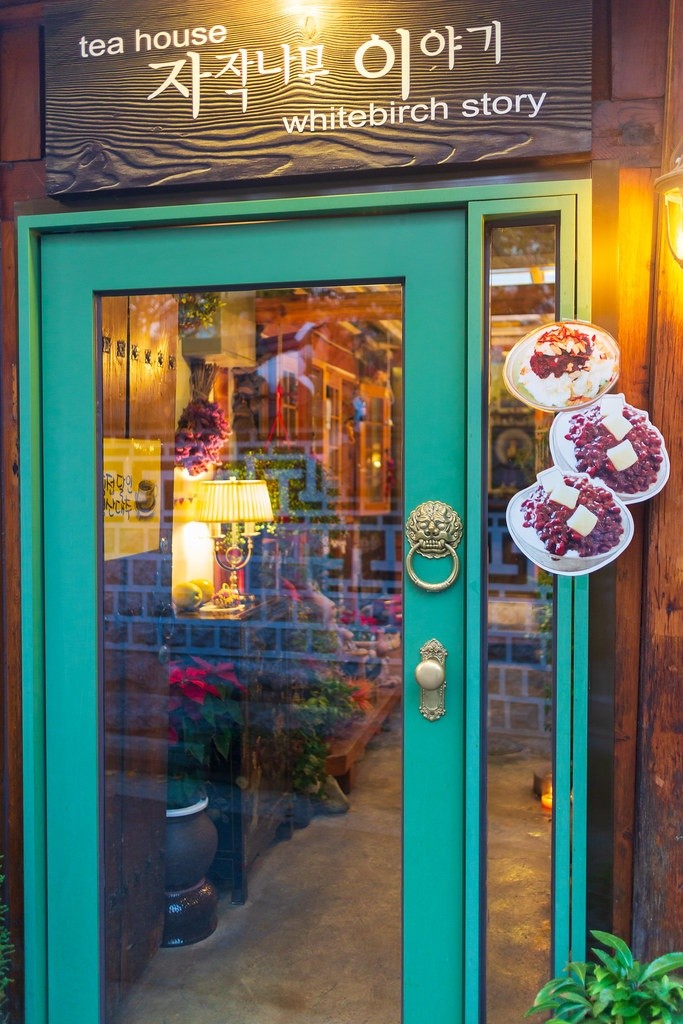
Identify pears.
[191,579,213,603]
[173,582,203,609]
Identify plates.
[506,480,635,577]
[503,321,620,411]
[549,398,670,506]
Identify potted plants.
[215,382,351,552]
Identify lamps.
[649,140,682,269]
[191,476,276,604]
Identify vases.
[177,317,200,335]
[164,791,219,892]
[354,641,377,672]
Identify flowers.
[176,292,227,329]
[166,655,251,810]
[174,360,234,476]
[340,610,376,640]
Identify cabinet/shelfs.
[268,350,396,533]
[170,596,298,907]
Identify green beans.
[522,479,623,555]
[565,406,662,493]
